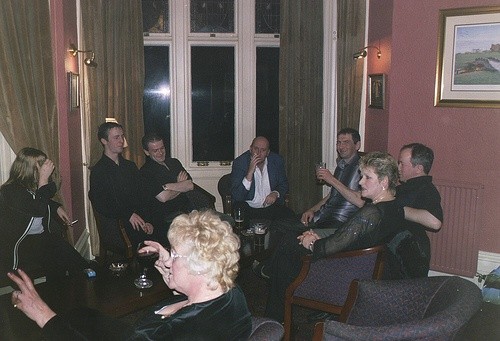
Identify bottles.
[317,162,326,184]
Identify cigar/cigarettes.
[254,153,257,156]
[302,222,308,223]
[69,220,78,226]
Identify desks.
[233,229,282,318]
[11,254,183,329]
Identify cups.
[139,273,152,288]
[254,223,267,234]
[235,208,246,222]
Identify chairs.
[311,275,484,341]
[0,209,70,292]
[282,242,393,341]
[217,172,290,231]
[244,315,285,341]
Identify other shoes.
[255,264,273,278]
[309,312,332,321]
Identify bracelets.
[164,184,168,190]
[309,240,315,252]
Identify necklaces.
[372,195,393,203]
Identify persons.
[0,122,443,324]
[7,210,252,341]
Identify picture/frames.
[432,5,500,109]
[67,72,80,114]
[367,72,387,110]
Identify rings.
[14,303,17,308]
[17,295,19,300]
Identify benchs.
[88,164,215,260]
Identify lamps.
[353,46,382,61]
[68,44,98,69]
